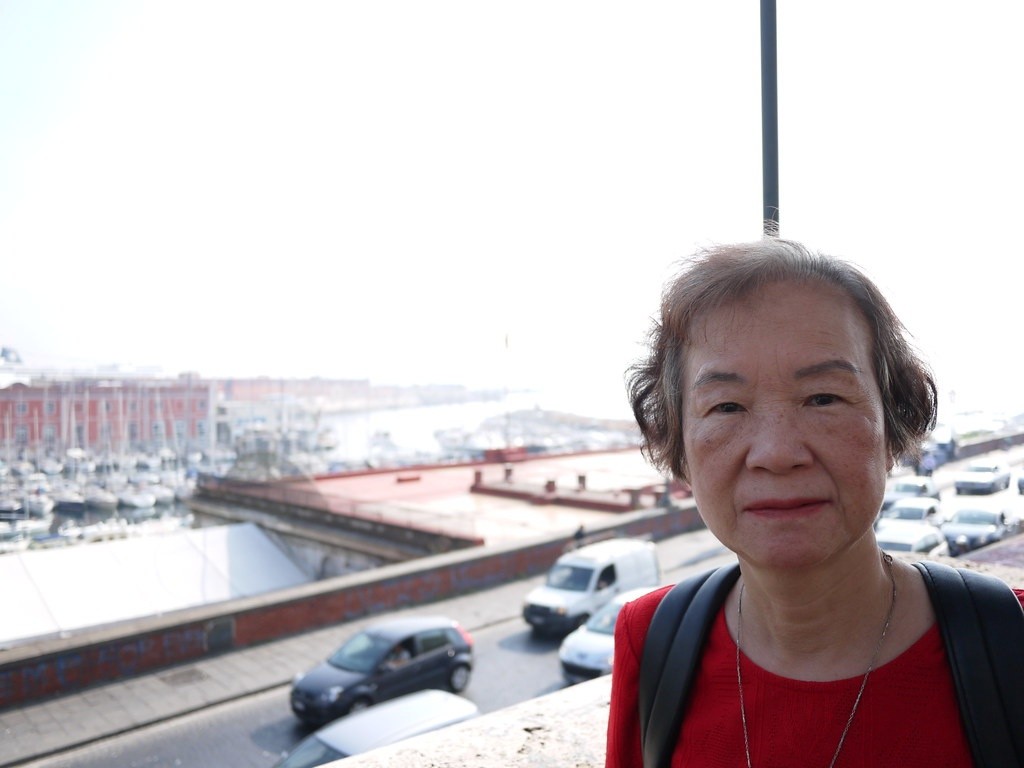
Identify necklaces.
[736,546,899,766]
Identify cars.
[875,451,1024,562]
[557,586,657,682]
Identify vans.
[523,540,661,635]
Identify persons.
[388,644,411,666]
[605,236,1024,768]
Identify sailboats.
[0,402,559,528]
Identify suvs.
[276,688,481,768]
[291,616,477,730]
